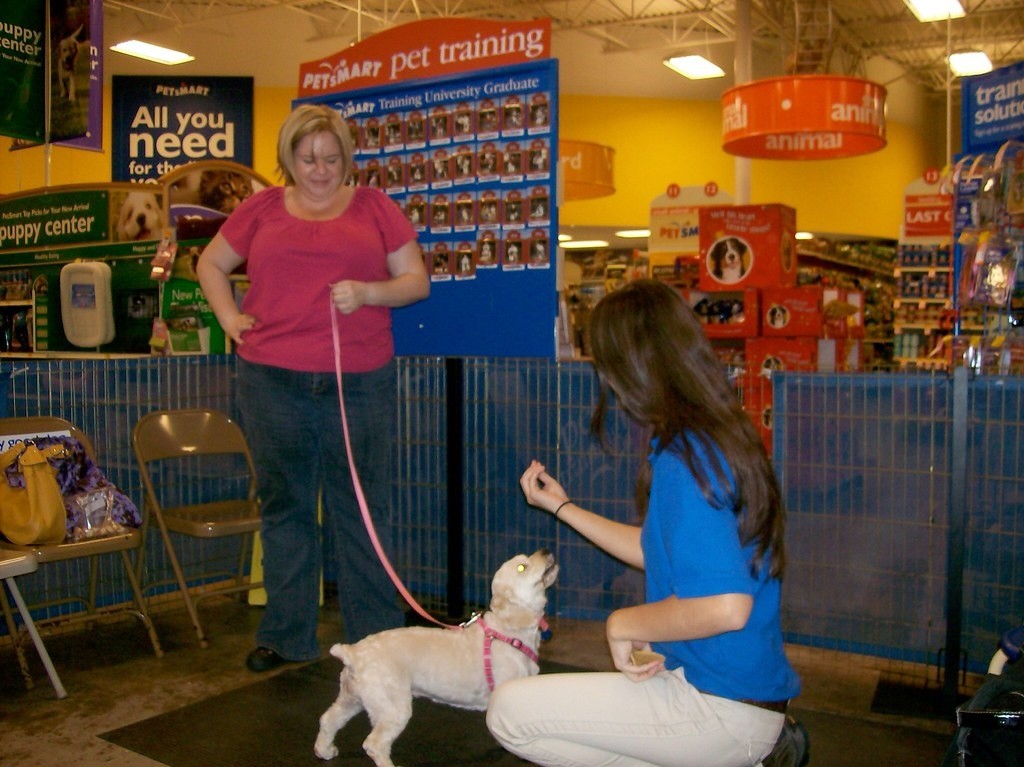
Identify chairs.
[0,549,70,697]
[133,409,261,650]
[0,414,162,692]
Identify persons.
[485,280,810,767]
[196,104,430,674]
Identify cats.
[169,169,254,216]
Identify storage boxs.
[653,200,865,467]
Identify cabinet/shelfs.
[888,242,1019,376]
[795,243,897,370]
[564,242,646,361]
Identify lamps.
[663,47,728,80]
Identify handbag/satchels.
[2,437,143,545]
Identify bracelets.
[554,500,573,516]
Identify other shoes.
[245,647,287,673]
[764,715,811,767]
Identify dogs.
[769,307,786,326]
[116,191,163,242]
[762,407,774,426]
[711,237,747,283]
[313,548,561,767]
[765,357,780,375]
